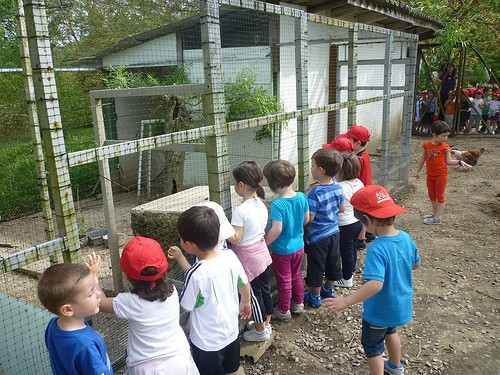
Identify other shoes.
[365,234,376,240]
[421,214,442,224]
[354,240,366,250]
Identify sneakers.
[383,358,404,375]
[320,283,337,298]
[290,301,304,314]
[334,274,353,287]
[272,305,291,322]
[243,326,270,341]
[246,320,273,334]
[303,292,322,308]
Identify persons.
[217,160,274,343]
[84,235,201,375]
[170,207,252,375]
[337,124,378,248]
[322,137,353,156]
[164,201,234,271]
[262,159,310,321]
[322,186,421,375]
[415,121,475,224]
[303,149,346,306]
[415,83,500,137]
[333,156,366,289]
[37,261,116,375]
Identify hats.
[193,201,236,240]
[120,236,169,281]
[466,88,473,96]
[322,137,353,153]
[474,88,484,95]
[350,185,408,219]
[338,125,371,142]
[463,90,469,95]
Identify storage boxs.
[87,229,107,246]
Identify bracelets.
[458,160,462,166]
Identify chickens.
[451,147,485,166]
